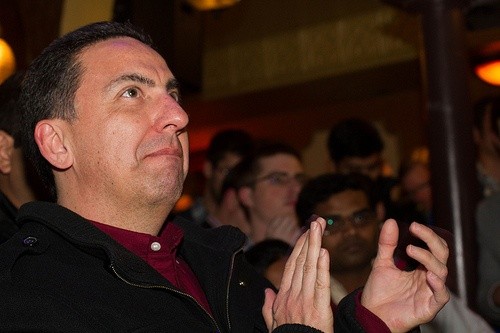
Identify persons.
[198,91,500,333]
[0,61,58,249]
[0,19,451,333]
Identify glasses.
[250,170,306,189]
[325,210,375,234]
